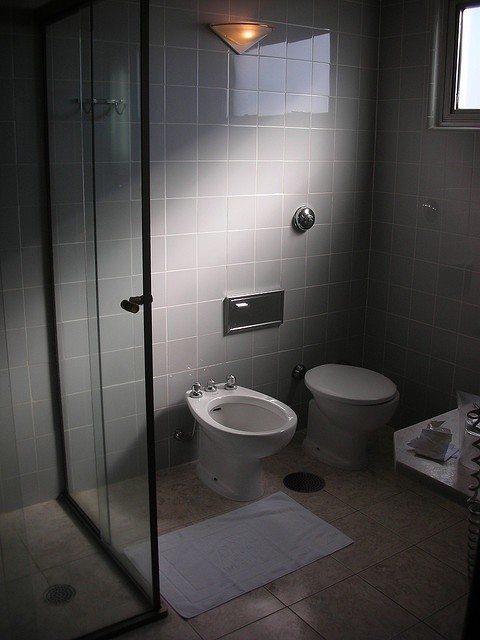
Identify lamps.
[210,19,273,55]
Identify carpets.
[123,489,355,619]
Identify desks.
[393,400,477,514]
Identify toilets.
[175,374,298,501]
[299,363,401,471]
